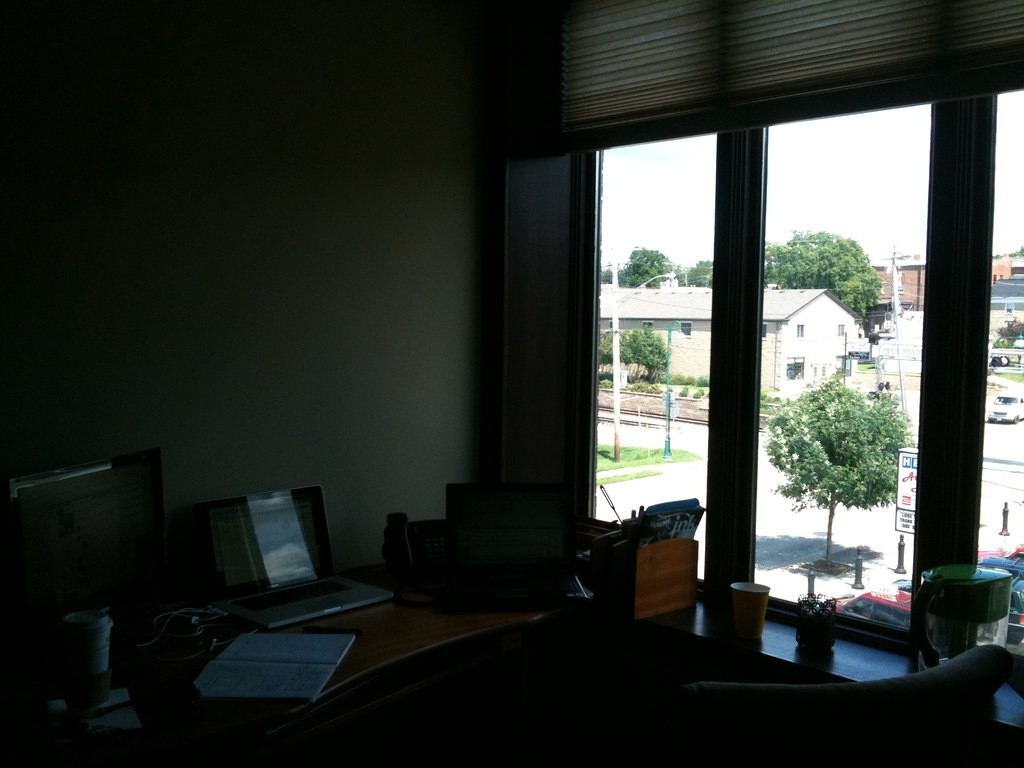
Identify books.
[194,633,355,701]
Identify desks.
[0,567,596,768]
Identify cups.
[729,582,770,639]
[59,610,114,674]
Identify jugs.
[910,564,1013,672]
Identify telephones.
[378,512,454,585]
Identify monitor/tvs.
[0,447,171,636]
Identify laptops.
[441,481,578,610]
[194,485,403,629]
